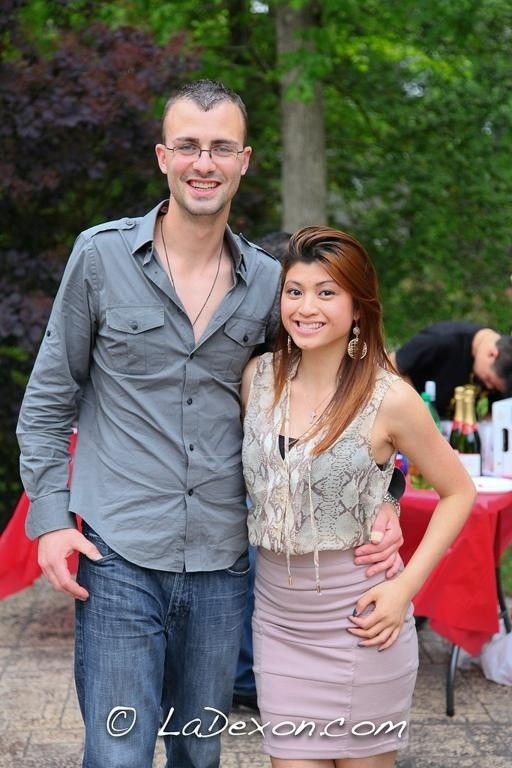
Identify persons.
[229,221,482,768]
[377,318,511,427]
[11,73,406,768]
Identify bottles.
[395,380,482,489]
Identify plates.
[469,476,512,494]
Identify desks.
[389,462,512,718]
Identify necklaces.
[156,214,227,330]
[298,372,338,424]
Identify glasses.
[164,142,244,162]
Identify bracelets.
[383,490,401,518]
